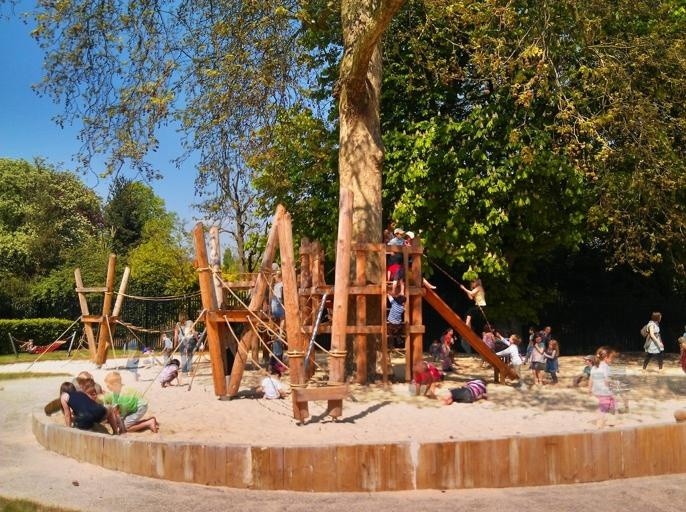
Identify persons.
[569,355,594,386]
[413,280,559,404]
[60,367,159,433]
[384,224,438,353]
[268,264,290,375]
[123,311,203,388]
[678,337,685,372]
[587,346,618,414]
[17,339,67,354]
[641,311,667,369]
